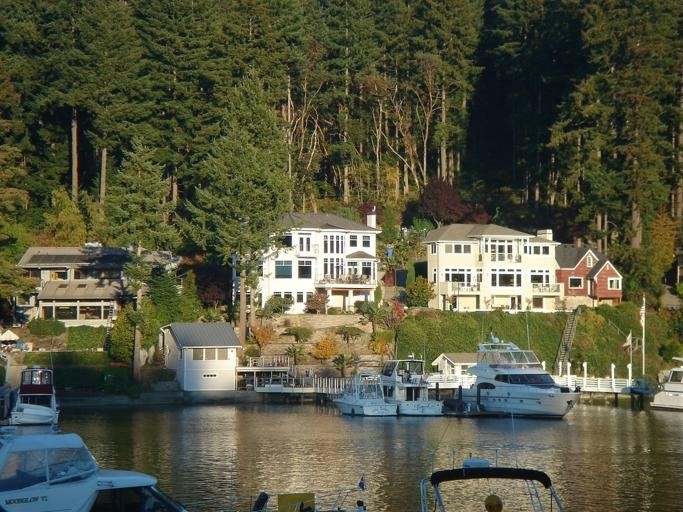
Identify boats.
[9,367,61,426]
[330,324,583,421]
[0,432,188,512]
[651,364,682,410]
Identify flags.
[622,333,632,346]
[639,299,645,327]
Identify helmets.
[485,495,502,512]
